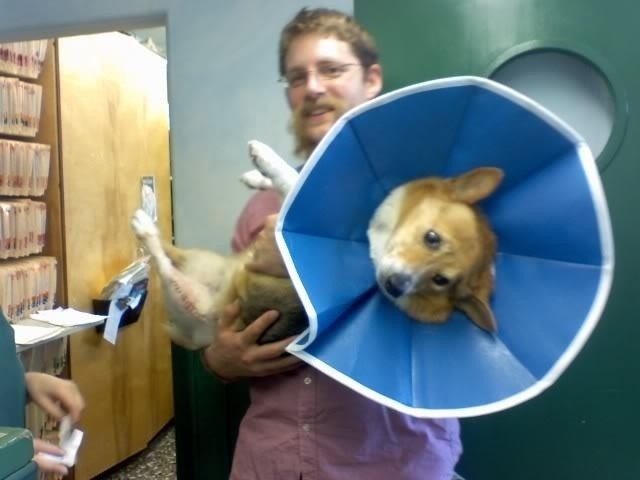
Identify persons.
[1,310,84,476]
[203,7,467,479]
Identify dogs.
[129,138,504,347]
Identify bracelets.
[204,351,233,382]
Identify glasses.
[277,60,365,89]
